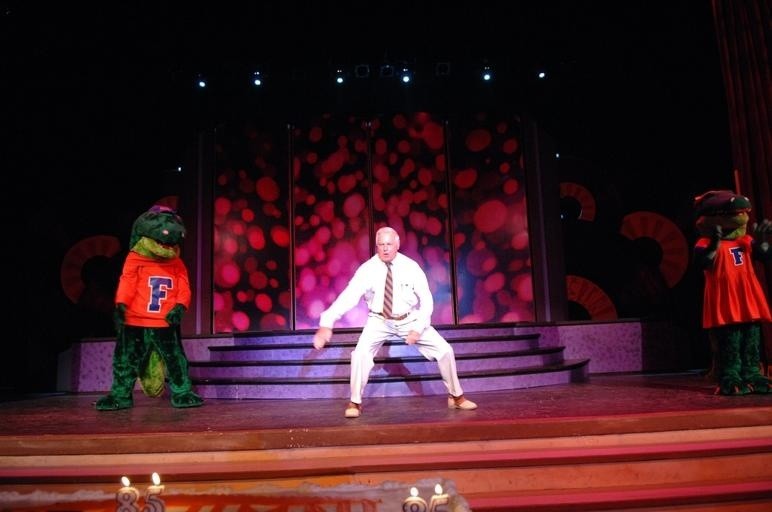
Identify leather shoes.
[447,393,478,410]
[344,402,362,418]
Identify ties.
[382,262,393,319]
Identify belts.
[373,312,409,320]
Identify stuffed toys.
[692,190,771,397]
[93,207,205,412]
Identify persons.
[313,226,478,419]
